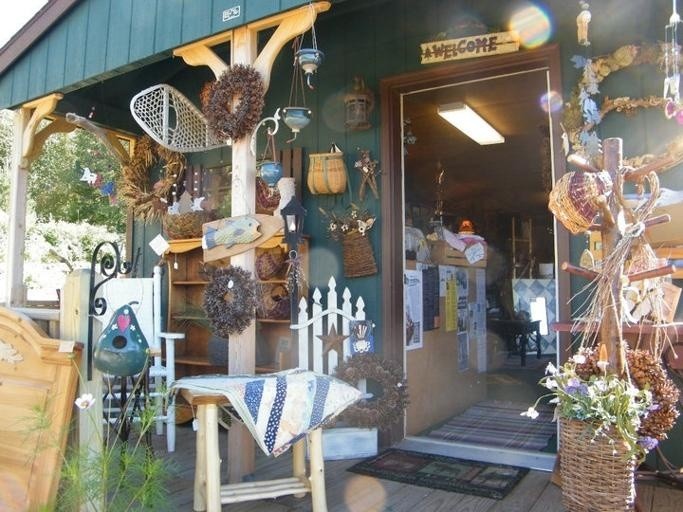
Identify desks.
[489,320,541,367]
[553,322,683,368]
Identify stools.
[180,388,328,512]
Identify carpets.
[427,400,556,452]
[345,448,530,501]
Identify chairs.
[102,265,185,452]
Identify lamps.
[437,102,505,146]
[344,75,375,131]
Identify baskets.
[307,153,347,195]
[558,415,636,512]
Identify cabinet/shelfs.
[162,234,310,424]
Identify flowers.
[520,355,659,464]
[319,202,376,242]
